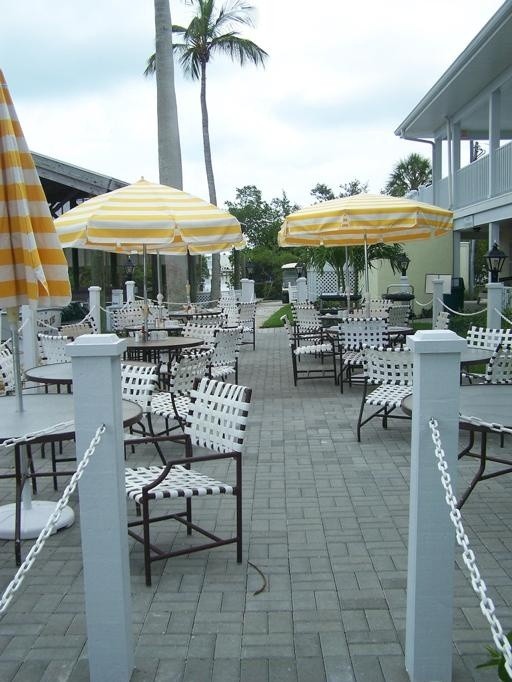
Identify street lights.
[294,256,314,297]
[391,251,413,302]
[474,239,511,331]
[242,258,258,298]
[119,255,138,302]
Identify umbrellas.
[1,66,74,414]
[272,186,456,316]
[51,174,248,334]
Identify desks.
[2,394,143,541]
[23,358,158,458]
[398,383,511,516]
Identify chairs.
[279,298,454,394]
[126,375,254,587]
[32,294,259,393]
[355,324,512,442]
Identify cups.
[149,331,168,340]
[338,310,349,318]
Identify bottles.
[154,317,159,328]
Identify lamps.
[123,256,136,280]
[483,241,508,282]
[395,254,410,276]
